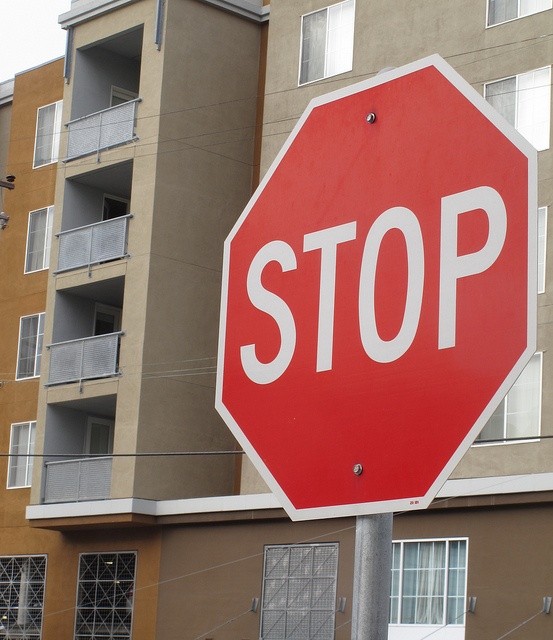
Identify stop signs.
[212,53,538,524]
